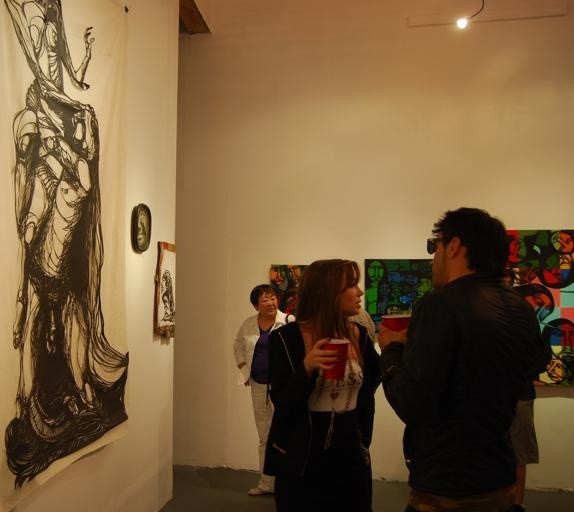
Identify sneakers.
[248,488,266,495]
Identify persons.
[233,259,377,511]
[136,205,149,253]
[10,21,104,425]
[378,208,553,511]
[160,271,176,325]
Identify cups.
[382,315,411,335]
[322,339,349,383]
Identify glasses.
[427,238,450,255]
